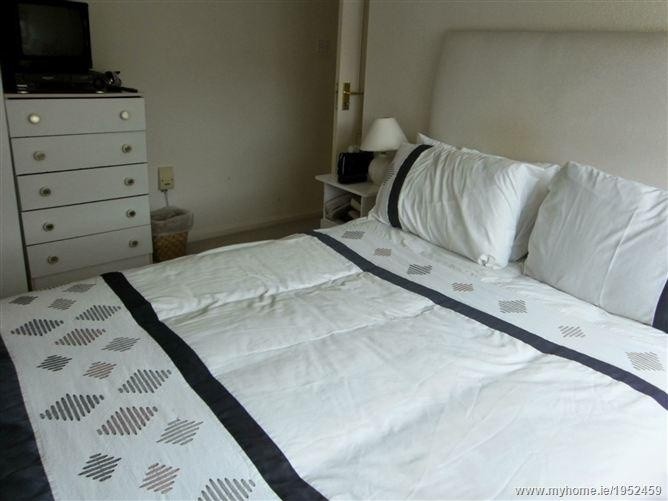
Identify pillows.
[367,130,559,272]
[521,162,668,335]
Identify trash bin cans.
[151,206,194,263]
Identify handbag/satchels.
[325,194,351,221]
[337,151,374,184]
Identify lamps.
[358,116,409,187]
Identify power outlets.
[158,166,174,194]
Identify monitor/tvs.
[0,0,93,93]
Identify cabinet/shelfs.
[1,92,154,301]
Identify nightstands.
[315,171,381,229]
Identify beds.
[0,212,668,500]
